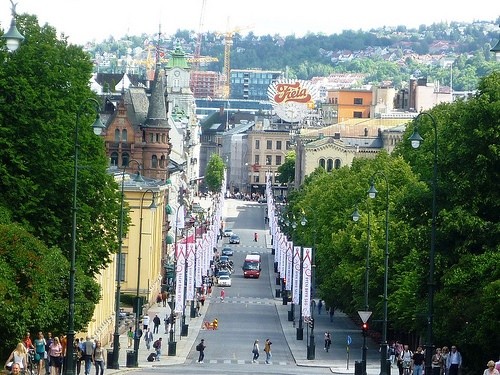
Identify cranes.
[131,43,220,81]
[211,22,258,101]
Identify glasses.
[490,366,494,367]
[451,348,455,349]
[54,339,57,341]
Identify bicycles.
[27,348,40,375]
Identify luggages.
[147,352,157,362]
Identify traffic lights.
[309,318,314,329]
[362,323,367,337]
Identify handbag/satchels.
[264,349,265,351]
[253,343,255,353]
[90,349,96,362]
[44,351,48,359]
[35,354,40,361]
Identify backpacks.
[153,340,159,348]
[196,343,201,351]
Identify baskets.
[403,361,410,369]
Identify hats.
[256,339,259,341]
[201,339,205,341]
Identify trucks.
[242,254,262,279]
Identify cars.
[217,275,232,287]
[229,235,240,245]
[221,247,235,256]
[251,251,261,262]
[217,256,231,278]
[223,230,234,237]
[258,197,267,203]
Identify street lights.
[167,202,194,356]
[291,211,304,340]
[108,159,146,370]
[284,212,295,321]
[181,190,220,337]
[130,189,158,368]
[406,113,439,375]
[351,198,369,375]
[265,177,288,306]
[300,206,315,360]
[63,98,107,375]
[366,172,392,375]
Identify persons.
[221,288,225,303]
[265,339,272,364]
[154,338,162,361]
[198,339,206,364]
[138,326,142,349]
[318,300,322,314]
[153,315,160,334]
[7,363,25,375]
[92,341,105,375]
[143,313,150,330]
[157,291,167,307]
[253,339,259,364]
[379,340,426,375]
[431,346,462,375]
[208,286,212,297]
[143,328,153,349]
[324,332,331,351]
[127,326,134,348]
[47,336,62,375]
[4,330,99,375]
[197,284,206,317]
[483,359,500,375]
[164,314,169,334]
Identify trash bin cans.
[125,349,136,367]
[276,277,279,285]
[106,349,113,368]
[283,297,287,305]
[296,328,304,339]
[272,249,274,255]
[73,352,77,375]
[274,263,276,268]
[275,289,280,297]
[168,342,177,356]
[355,361,363,375]
[307,345,315,358]
[380,359,392,375]
[265,219,268,224]
[194,308,196,316]
[185,325,188,336]
[288,311,292,320]
[210,260,213,267]
[196,287,200,292]
[207,270,210,276]
